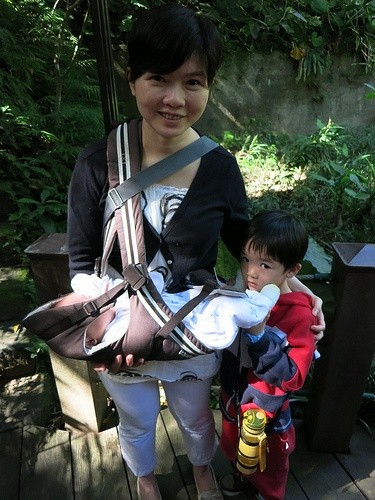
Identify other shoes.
[218,469,266,500]
[192,463,224,500]
[136,476,162,500]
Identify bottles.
[236,408,269,475]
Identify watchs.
[244,329,265,345]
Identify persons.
[45,273,281,359]
[60,3,325,500]
[219,206,320,500]
